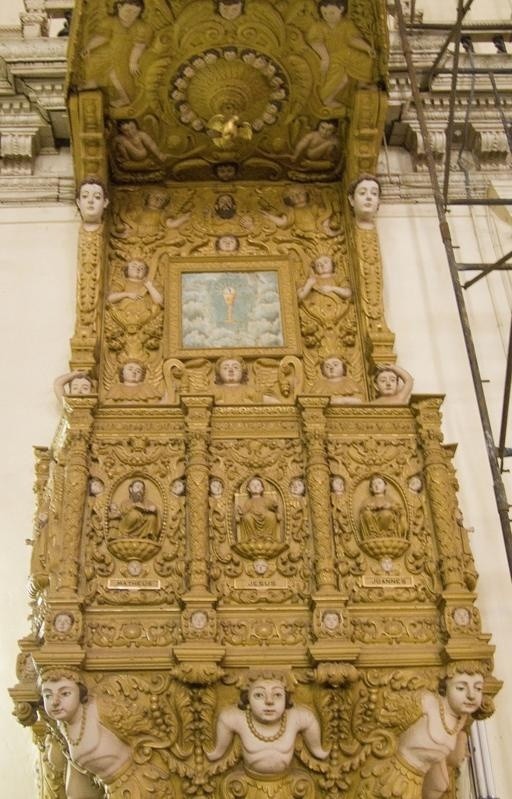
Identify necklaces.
[435,693,461,736]
[244,705,288,743]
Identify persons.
[21,666,158,797]
[59,706,91,745]
[358,657,485,799]
[193,668,330,798]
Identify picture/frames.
[159,250,301,364]
